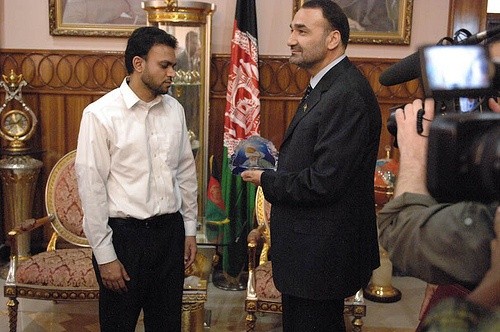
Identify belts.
[108,211,179,224]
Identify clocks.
[0,69,43,267]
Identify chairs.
[6,149,208,332]
[245,185,367,332]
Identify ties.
[298,82,313,107]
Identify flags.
[223,0,261,142]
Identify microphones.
[381,26,499,85]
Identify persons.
[74,27,198,332]
[378,97,500,332]
[171,31,199,129]
[241,0,383,332]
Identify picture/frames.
[293,0,414,46]
[48,0,159,37]
